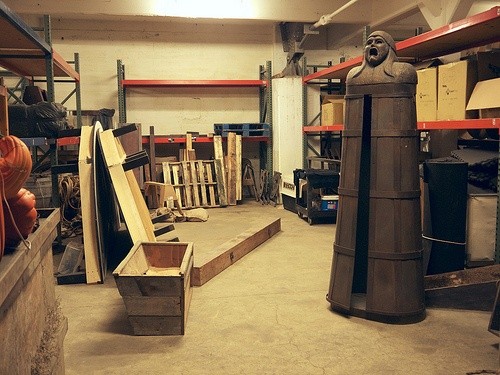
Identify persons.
[347,31,418,84]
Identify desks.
[0,207,68,375]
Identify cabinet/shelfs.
[301,6,500,270]
[116,59,273,195]
[0,0,81,246]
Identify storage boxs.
[321,194,340,210]
[321,94,345,126]
[416,47,500,121]
[281,177,307,213]
[112,242,194,336]
